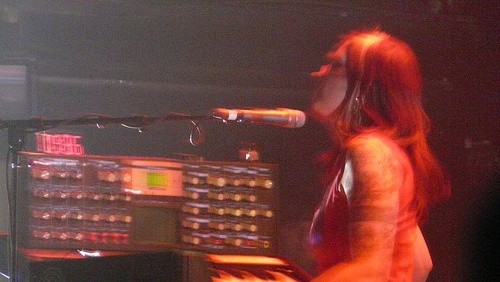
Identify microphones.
[212,108,306,129]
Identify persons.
[308,30,450,282]
